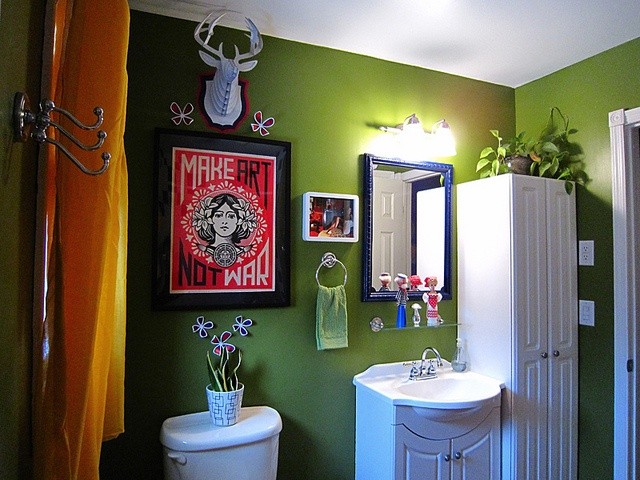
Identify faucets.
[418,345,443,377]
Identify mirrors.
[361,152,453,302]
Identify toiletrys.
[450,335,469,372]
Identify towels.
[315,284,348,350]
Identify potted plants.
[206,344,245,427]
[475,107,577,196]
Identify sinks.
[353,358,506,422]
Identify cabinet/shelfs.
[457,175,578,480]
[355,393,503,480]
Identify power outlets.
[579,240,594,266]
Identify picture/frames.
[302,192,359,243]
[149,128,292,310]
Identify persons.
[395,273,410,329]
[422,276,443,322]
[192,193,257,256]
[324,204,334,228]
